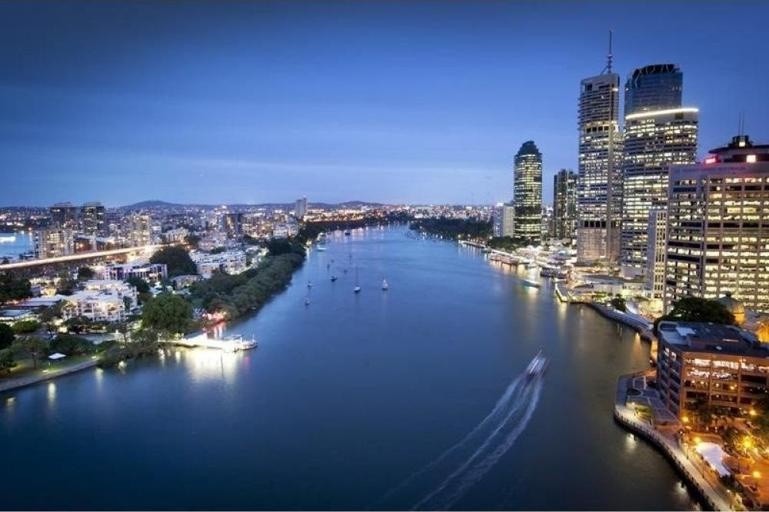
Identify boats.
[303,245,389,308]
[236,337,258,351]
[344,230,350,236]
[490,253,516,264]
[522,277,541,287]
[526,349,545,376]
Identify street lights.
[680,399,762,508]
